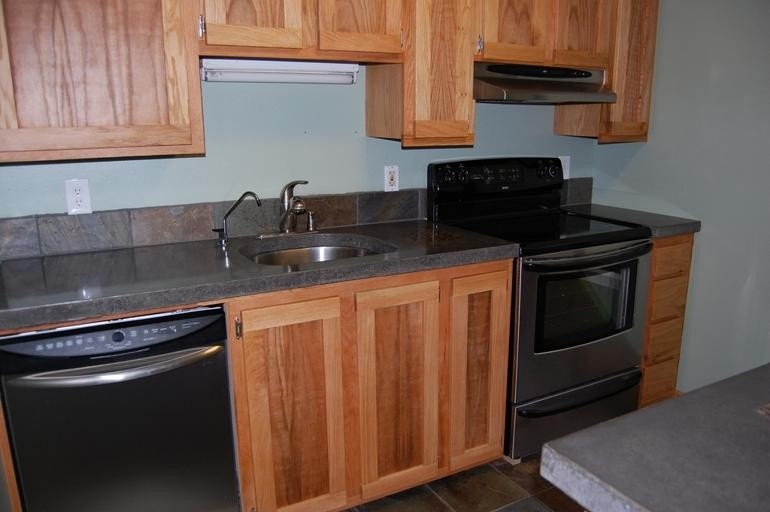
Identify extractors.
[473,60,619,104]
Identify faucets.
[278,180,309,235]
[222,191,262,245]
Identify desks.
[538,360,770,510]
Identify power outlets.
[382,164,403,193]
[65,177,93,216]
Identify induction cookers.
[444,210,650,258]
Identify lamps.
[200,56,359,87]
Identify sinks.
[237,233,399,266]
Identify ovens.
[503,240,652,464]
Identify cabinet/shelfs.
[639,232,694,408]
[366,1,475,149]
[230,259,514,512]
[555,1,661,145]
[1,1,206,176]
[201,1,405,68]
[476,1,614,71]
